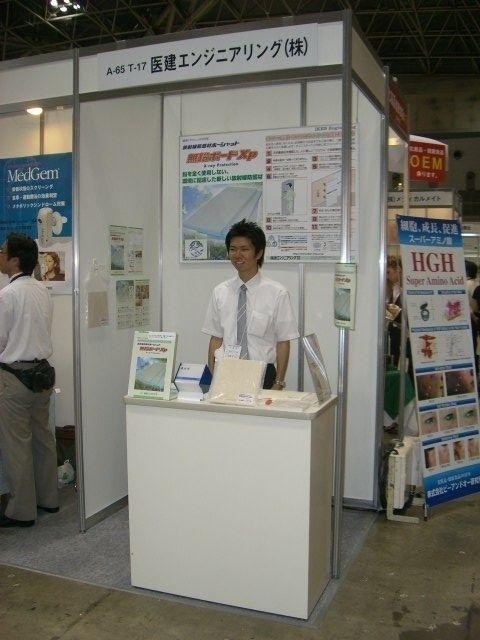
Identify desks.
[123,388,338,621]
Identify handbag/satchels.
[15,360,55,393]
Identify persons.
[384,253,416,383]
[416,367,480,468]
[200,217,301,394]
[470,284,479,337]
[41,252,65,281]
[0,230,62,528]
[464,259,479,354]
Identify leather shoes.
[37,505,59,513]
[0,514,34,527]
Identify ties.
[236,285,248,359]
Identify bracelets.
[273,379,287,388]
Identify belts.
[14,360,43,364]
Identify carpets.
[1,484,381,630]
